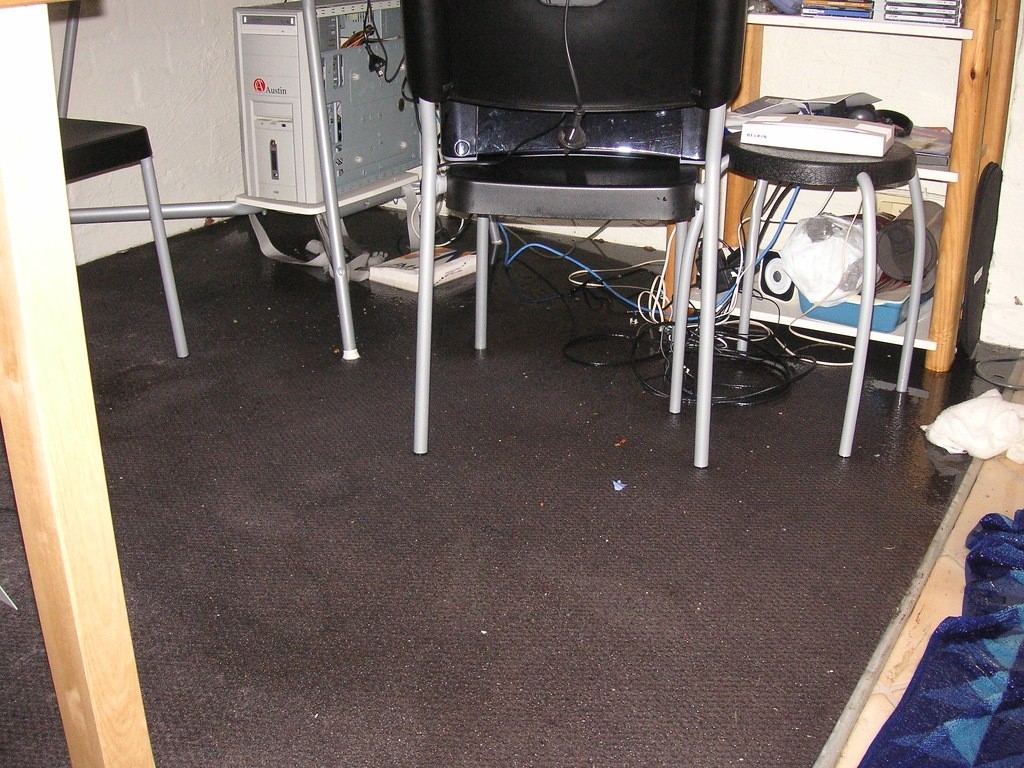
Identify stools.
[665,130,926,473]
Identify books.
[801,0,874,19]
[369,246,476,293]
[883,0,959,26]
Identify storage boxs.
[798,284,935,333]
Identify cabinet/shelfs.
[663,0,1024,372]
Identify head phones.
[848,104,914,138]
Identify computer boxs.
[232,0,421,206]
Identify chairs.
[401,0,746,457]
[57,0,191,358]
